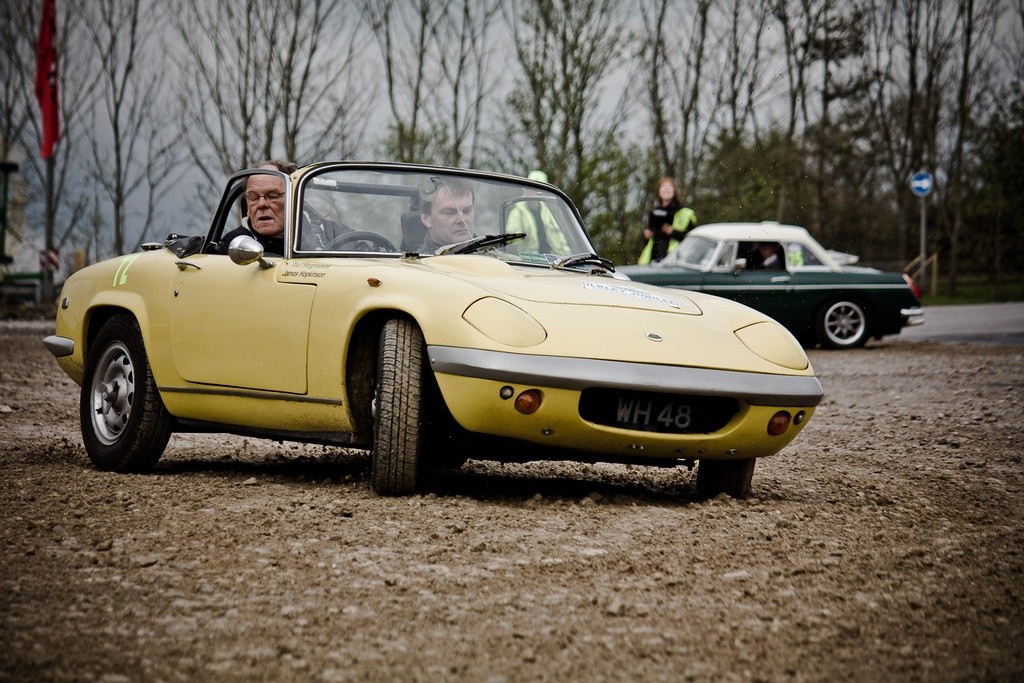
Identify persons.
[219,162,361,257]
[643,177,695,266]
[756,243,783,269]
[413,178,478,254]
[506,170,572,255]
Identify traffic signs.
[911,172,931,198]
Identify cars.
[614,222,923,347]
[42,162,826,498]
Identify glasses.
[245,190,285,201]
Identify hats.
[527,170,548,184]
